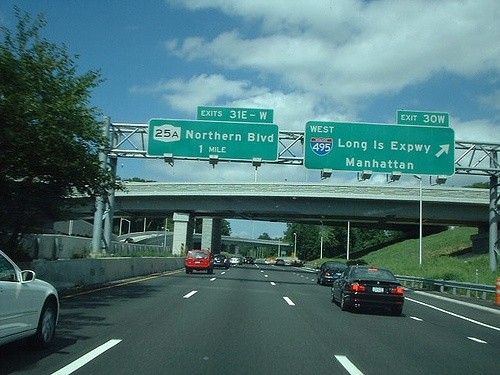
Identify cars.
[0,251,61,346]
[316,262,346,285]
[214,252,306,268]
[184,250,215,272]
[330,263,404,315]
